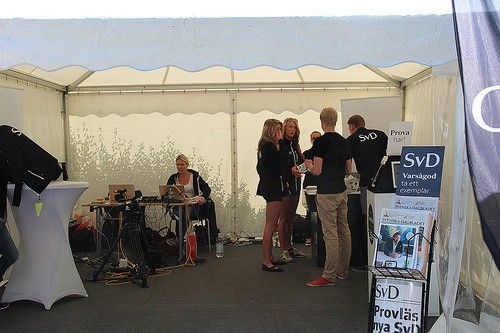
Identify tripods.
[93,205,158,288]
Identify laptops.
[109,183,140,201]
[159,184,194,201]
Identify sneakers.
[288,247,306,257]
[307,276,335,286]
[280,250,293,262]
[334,271,347,279]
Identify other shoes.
[271,259,287,265]
[304,238,311,246]
[0,302,9,310]
[262,264,284,272]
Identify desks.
[1,182,89,309]
[82,201,197,260]
[302,189,363,268]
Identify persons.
[304,106,352,287]
[278,118,306,262]
[0,214,19,309]
[256,119,304,272]
[346,114,388,273]
[166,155,219,256]
[303,131,322,177]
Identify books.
[396,145,445,197]
[387,121,412,156]
[371,195,439,279]
[372,278,423,333]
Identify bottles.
[216,233,224,258]
